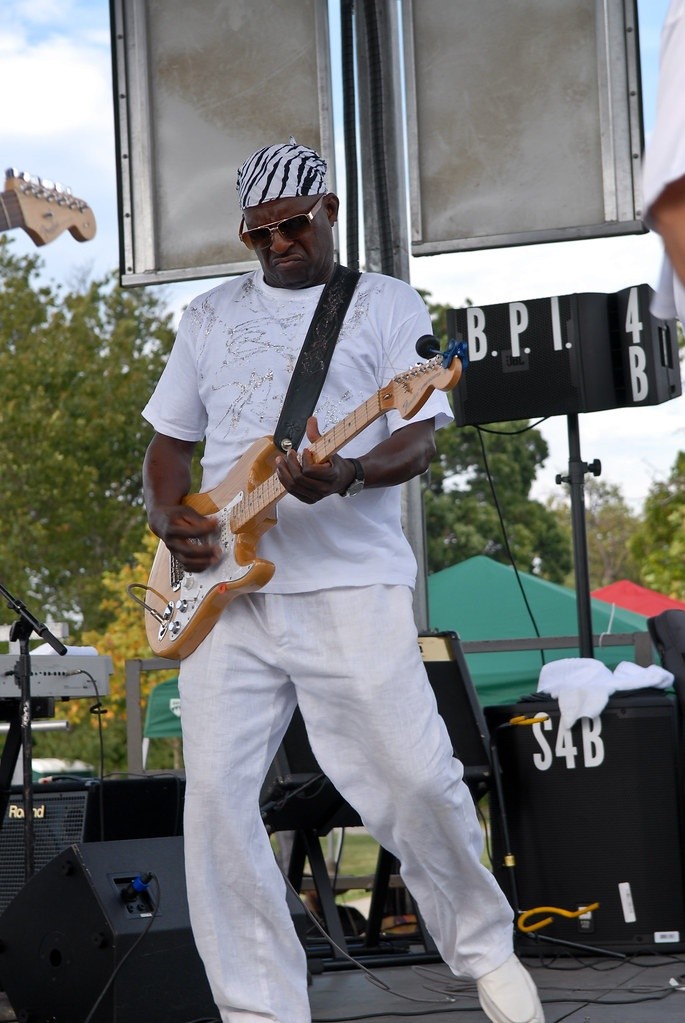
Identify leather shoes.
[476,954,545,1023]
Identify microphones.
[416,334,445,360]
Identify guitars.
[1,166,97,248]
[126,350,464,663]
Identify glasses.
[238,195,326,250]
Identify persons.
[139,137,547,1023]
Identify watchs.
[340,457,366,499]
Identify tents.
[143,556,675,821]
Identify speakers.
[446,282,680,427]
[0,772,223,1023]
[260,628,496,805]
[482,692,685,957]
